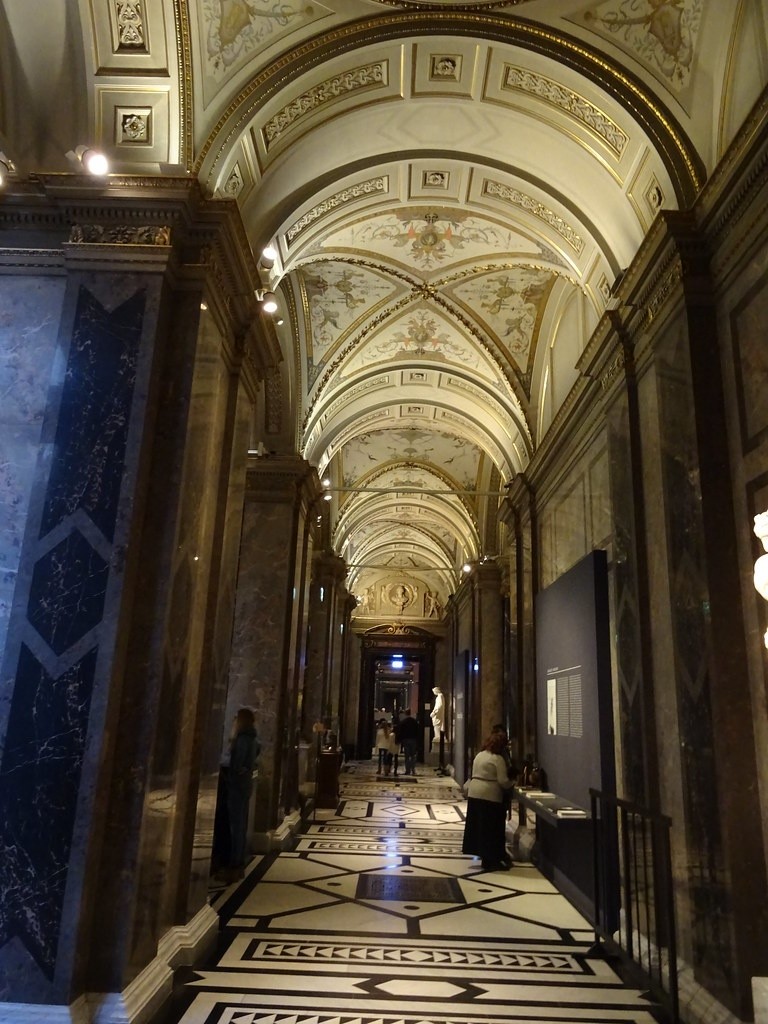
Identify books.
[517,785,587,819]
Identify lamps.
[463,555,489,571]
[259,246,281,316]
[66,143,110,180]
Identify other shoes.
[482,858,512,870]
[394,770,398,776]
[403,772,410,775]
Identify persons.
[462,725,518,871]
[430,687,443,743]
[214,709,260,882]
[374,706,421,777]
[314,723,335,748]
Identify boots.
[384,766,388,776]
[376,766,381,774]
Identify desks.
[315,751,344,808]
